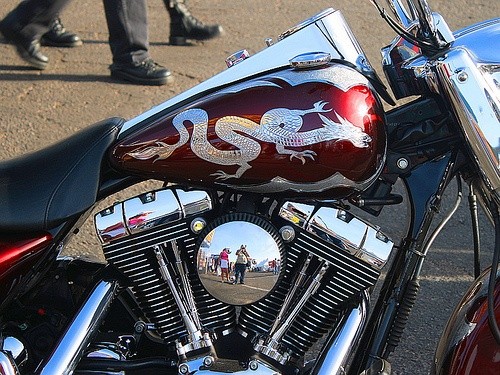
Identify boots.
[40,13,83,46]
[163,0,221,45]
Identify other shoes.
[227,280,231,282]
[222,281,225,282]
[233,282,236,284]
[240,282,244,284]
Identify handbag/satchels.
[216,265,222,276]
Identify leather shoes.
[0,21,49,69]
[112,58,173,85]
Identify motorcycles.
[0,0,500,375]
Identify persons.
[207,244,281,285]
[40,0,225,48]
[0,0,173,84]
